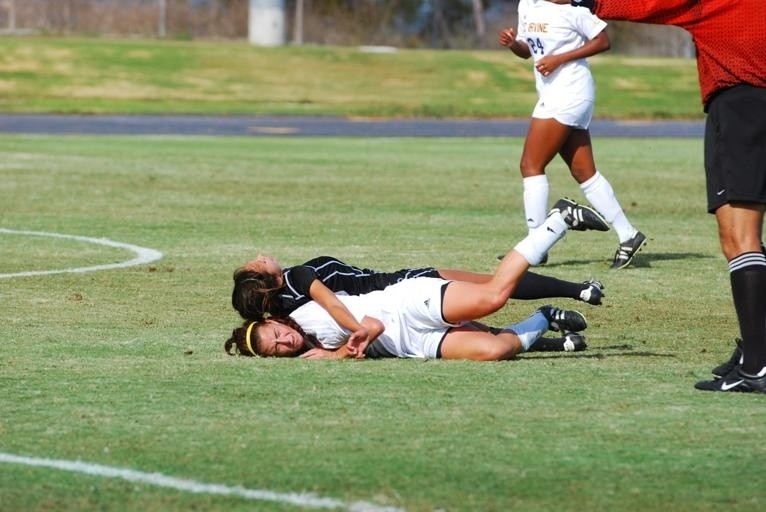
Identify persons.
[541,0,765,392]
[225,197,611,364]
[229,253,604,351]
[498,0,648,270]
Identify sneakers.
[695,336,766,391]
[579,276,604,303]
[532,305,588,352]
[610,231,647,270]
[547,195,609,232]
